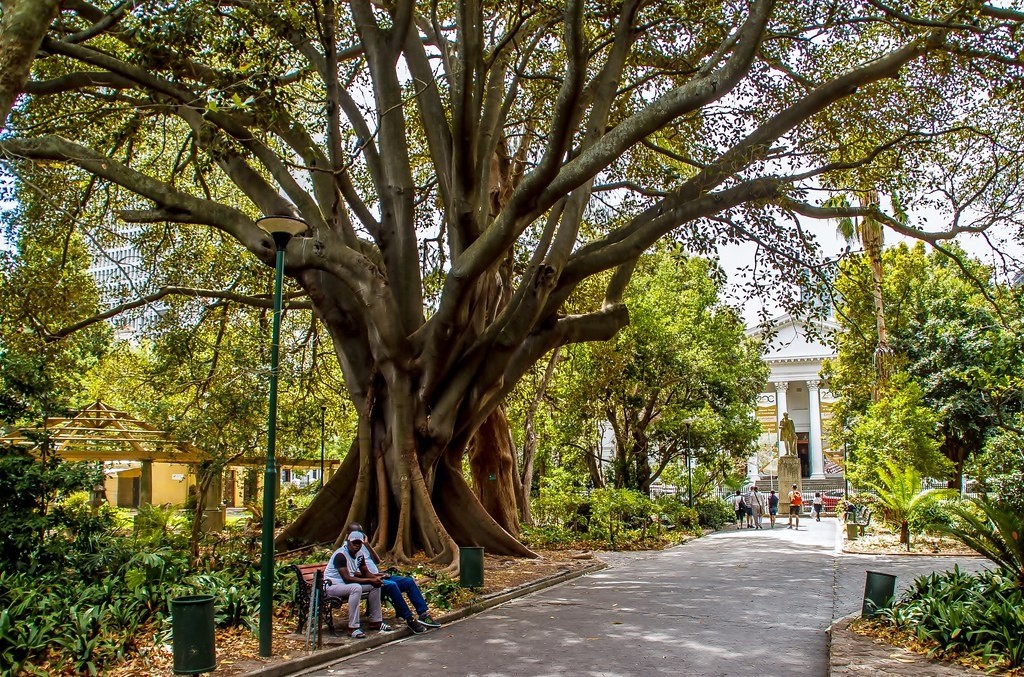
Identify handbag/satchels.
[760,506,765,515]
[769,507,778,513]
[811,508,817,516]
[739,497,747,513]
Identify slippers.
[369,623,392,630]
[348,629,365,638]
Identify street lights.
[320,406,327,487]
[254,215,311,659]
[682,419,695,508]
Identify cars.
[724,493,744,506]
[802,489,845,512]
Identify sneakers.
[417,613,442,627]
[407,618,427,634]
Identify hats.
[347,531,365,544]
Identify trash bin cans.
[862,570,896,619]
[458,546,485,588]
[847,524,857,540]
[171,595,216,675]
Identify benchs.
[287,563,401,634]
[845,505,874,536]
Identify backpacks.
[791,492,803,505]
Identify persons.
[768,489,778,528]
[750,486,765,529]
[322,531,391,638]
[344,522,441,634]
[744,486,755,528]
[813,492,822,521]
[733,490,746,529]
[288,499,297,509]
[779,412,795,456]
[788,484,802,530]
